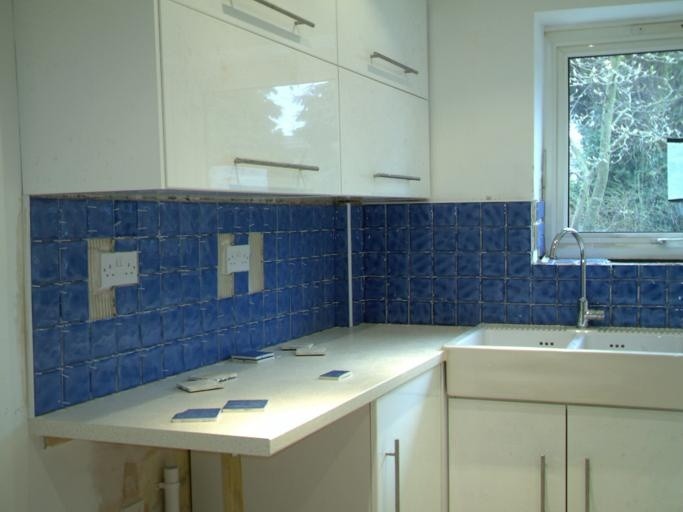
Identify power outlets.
[102,252,138,287]
[226,244,250,274]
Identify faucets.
[549,228,605,329]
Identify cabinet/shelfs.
[447,397,683,511]
[190,362,443,511]
[15,0,432,202]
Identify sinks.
[444,324,578,353]
[573,326,683,359]
